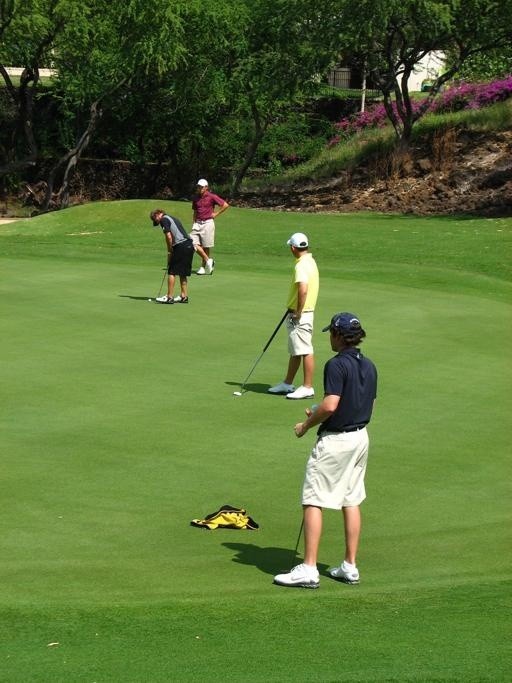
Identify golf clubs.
[278,519,304,574]
[233,310,289,395]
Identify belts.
[287,306,317,314]
[322,423,372,435]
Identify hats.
[151,208,164,226]
[321,312,362,333]
[286,231,309,249]
[196,179,209,187]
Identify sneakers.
[267,381,295,394]
[285,384,315,400]
[328,562,362,586]
[155,295,189,304]
[274,563,320,589]
[196,258,215,275]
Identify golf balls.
[311,405,319,412]
[148,299,152,302]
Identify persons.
[273,311,377,588]
[149,206,194,304]
[266,231,320,399]
[186,177,230,275]
